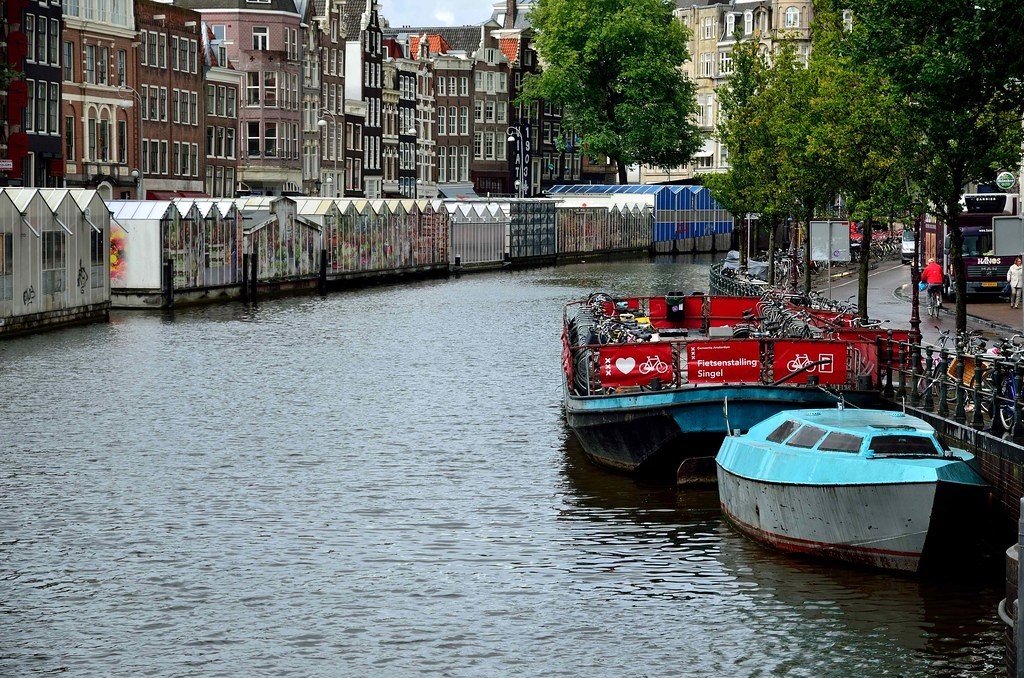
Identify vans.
[902,230,922,264]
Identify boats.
[713,403,992,579]
[561,291,886,487]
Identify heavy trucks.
[921,193,1024,302]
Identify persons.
[1006,257,1024,309]
[921,258,943,316]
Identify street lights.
[316,108,337,198]
[507,126,524,198]
[409,117,426,198]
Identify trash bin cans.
[665,291,686,323]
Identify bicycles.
[922,280,942,319]
[565,294,675,392]
[723,236,1024,433]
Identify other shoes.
[1015,307,1018,309]
[1011,302,1013,309]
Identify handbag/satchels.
[1002,282,1011,294]
[918,281,928,291]
